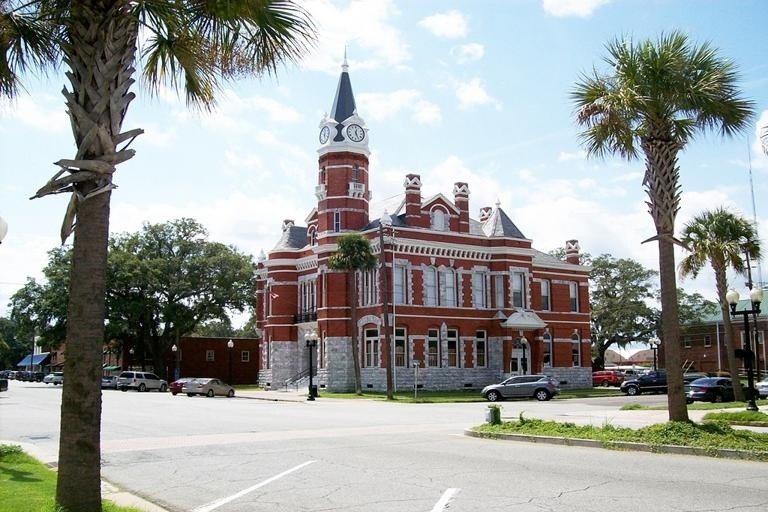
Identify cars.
[0,370,235,398]
[592,367,768,404]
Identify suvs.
[480,374,562,402]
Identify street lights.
[647,335,661,370]
[128,347,134,371]
[171,343,177,380]
[518,335,528,375]
[226,338,235,385]
[303,329,318,401]
[725,283,765,412]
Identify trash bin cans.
[0,379,8,391]
[307,385,317,400]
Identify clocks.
[319,126,330,144]
[346,123,365,142]
